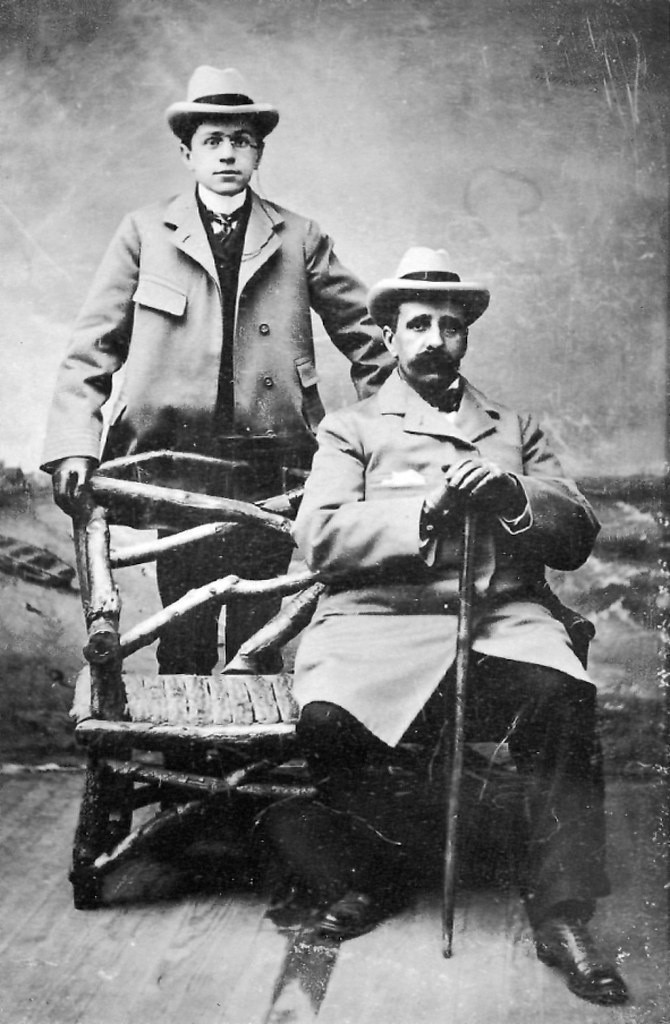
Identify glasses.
[192,137,259,151]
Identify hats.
[166,65,278,140]
[366,247,491,332]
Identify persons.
[41,64,394,674]
[297,244,626,1005]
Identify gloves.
[422,485,489,540]
[447,457,516,511]
[51,455,94,517]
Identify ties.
[211,212,234,245]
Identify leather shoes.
[319,881,416,935]
[537,916,629,1002]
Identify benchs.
[63,448,614,916]
